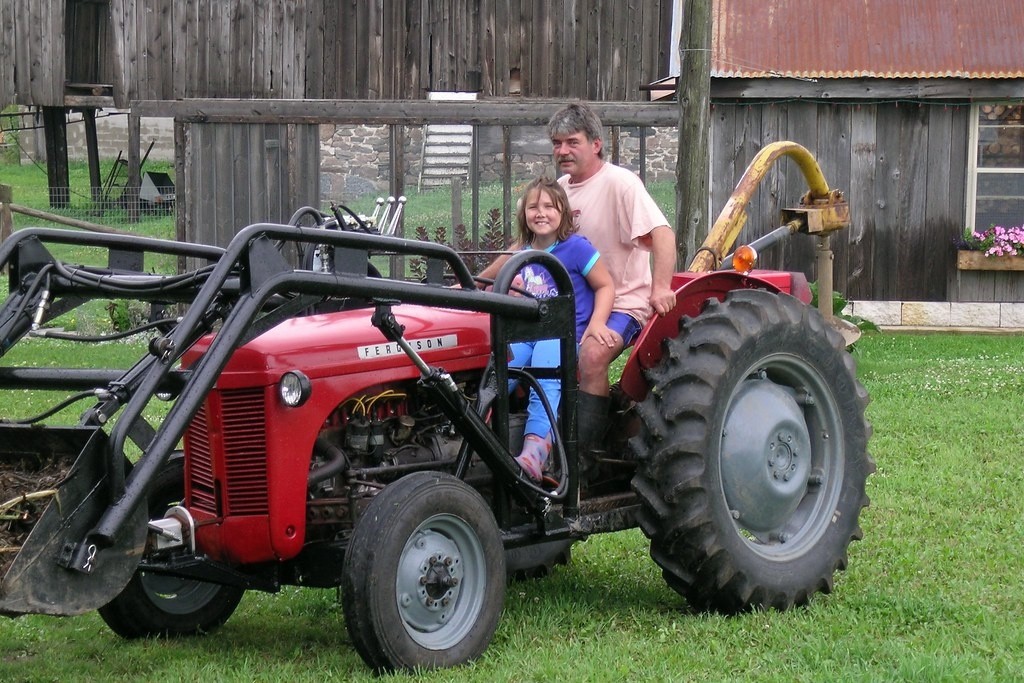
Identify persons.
[507,176,617,480]
[442,102,677,480]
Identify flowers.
[951,223,1024,258]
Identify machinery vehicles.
[0,135,877,676]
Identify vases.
[957,250,1024,271]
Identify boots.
[510,427,551,485]
[571,391,613,497]
